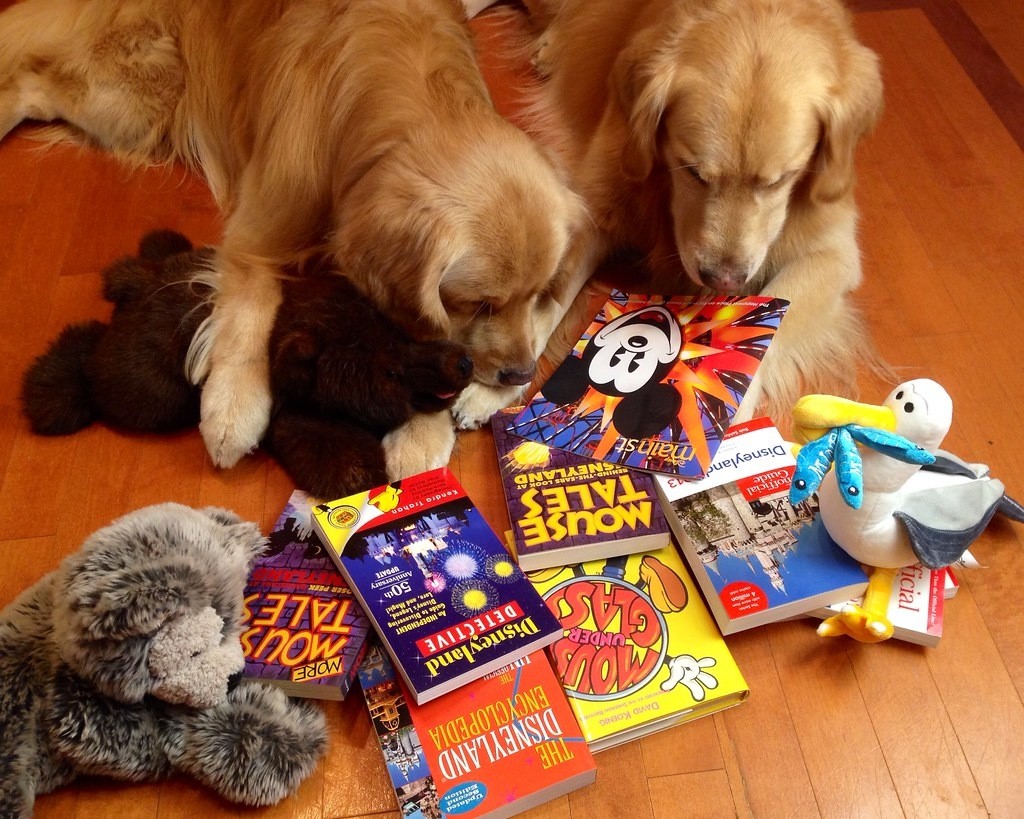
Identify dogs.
[447,0,930,432]
[0,0,599,486]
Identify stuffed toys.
[790,378,1024,645]
[0,500,327,819]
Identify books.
[649,417,959,648]
[491,411,672,571]
[357,621,597,819]
[504,528,750,755]
[310,467,565,705]
[229,487,375,703]
[507,289,791,479]
